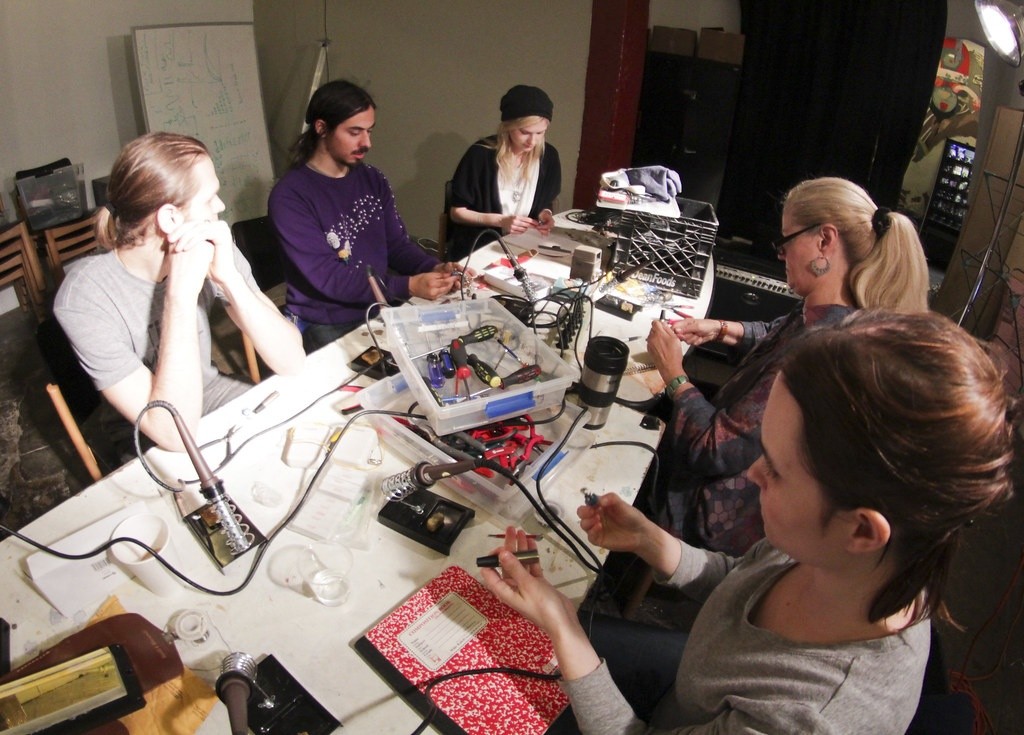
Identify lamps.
[958,0,1024,328]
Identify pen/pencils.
[660,309,666,322]
[620,336,643,342]
[538,245,572,253]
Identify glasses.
[770,221,824,256]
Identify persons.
[273,80,477,355]
[650,175,931,558]
[445,86,560,264]
[54,131,308,450]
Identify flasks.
[576,336,630,430]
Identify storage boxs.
[17,162,87,231]
[697,27,745,64]
[355,372,592,527]
[483,265,551,302]
[20,500,150,619]
[594,194,720,299]
[652,25,698,56]
[380,297,579,438]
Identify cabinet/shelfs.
[631,50,742,215]
[918,137,976,268]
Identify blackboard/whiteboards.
[132,22,277,228]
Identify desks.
[0,207,715,735]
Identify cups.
[110,512,181,595]
[298,539,353,606]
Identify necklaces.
[481,311,1024,735]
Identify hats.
[499,84,553,123]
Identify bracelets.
[665,375,691,399]
[715,320,728,342]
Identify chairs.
[904,625,973,735]
[437,180,453,263]
[15,156,104,292]
[37,315,120,482]
[231,213,289,384]
[0,196,46,325]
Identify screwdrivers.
[438,331,455,379]
[450,339,472,401]
[410,326,498,360]
[466,354,502,388]
[426,332,445,388]
[457,364,541,402]
